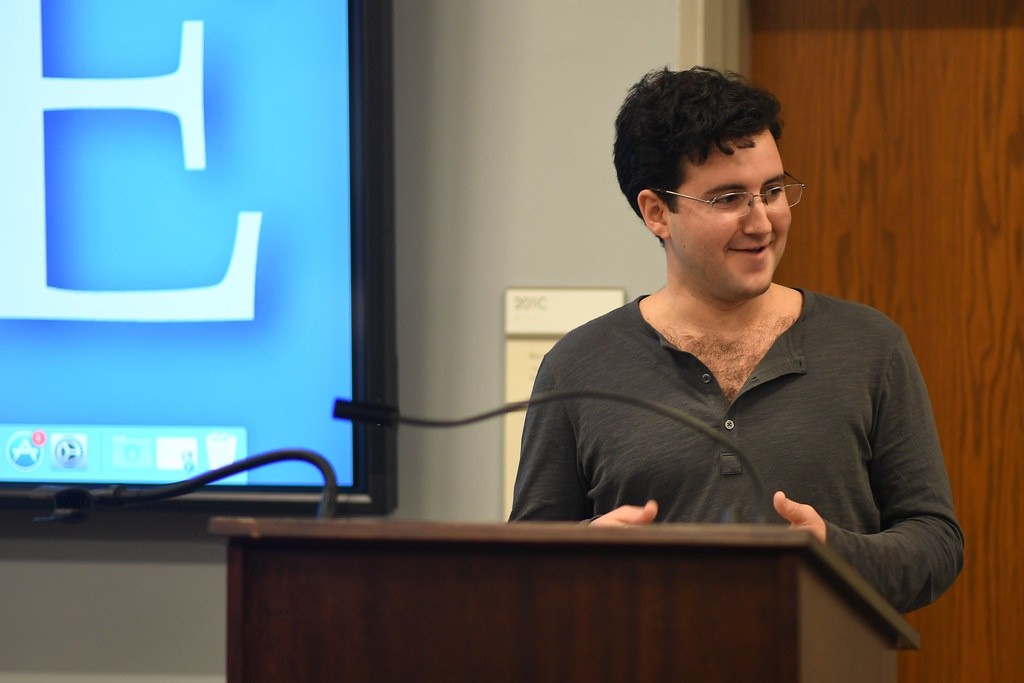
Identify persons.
[508,68,964,611]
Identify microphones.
[53,445,339,524]
[332,386,775,525]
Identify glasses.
[649,172,806,222]
